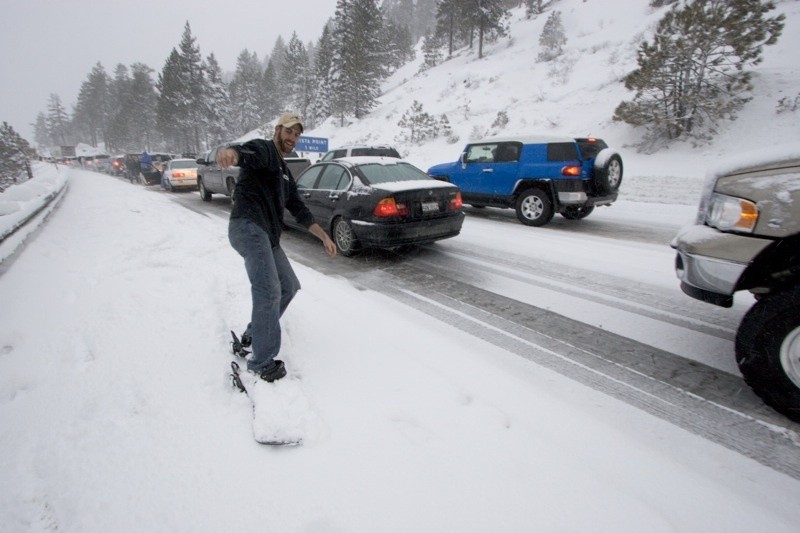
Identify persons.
[125,155,140,183]
[141,152,153,185]
[216,113,337,382]
[492,147,499,160]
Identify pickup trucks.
[196,141,312,205]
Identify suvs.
[314,145,402,159]
[673,160,799,426]
[424,138,624,227]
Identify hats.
[279,112,304,133]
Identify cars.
[42,151,196,185]
[160,158,198,191]
[280,157,465,256]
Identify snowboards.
[232,318,305,445]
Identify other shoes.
[241,335,252,347]
[247,358,277,374]
